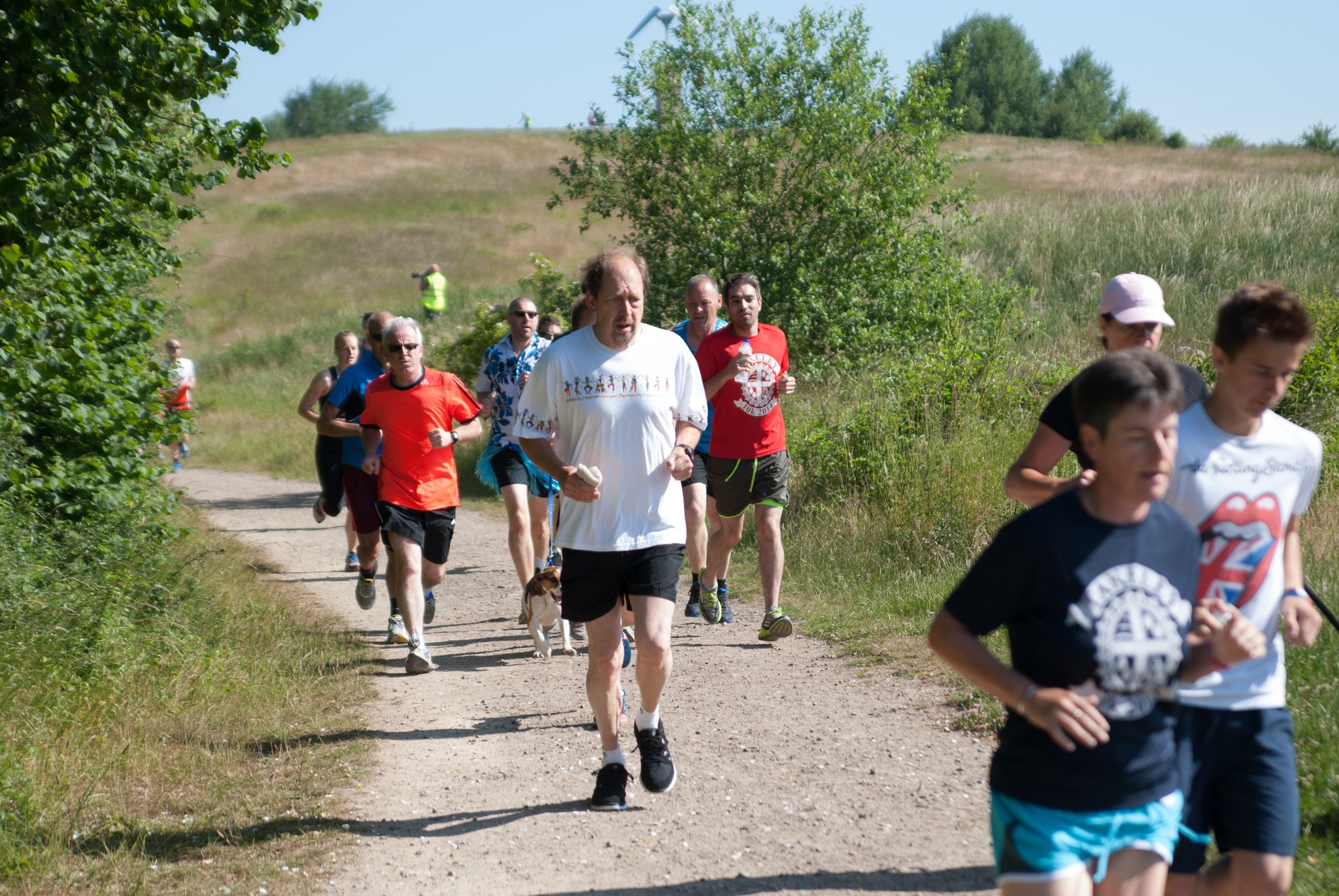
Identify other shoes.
[179,443,188,455]
[172,462,180,472]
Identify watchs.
[672,444,694,462]
[449,431,460,444]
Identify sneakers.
[387,615,410,643]
[313,492,326,523]
[698,565,721,624]
[354,560,378,610]
[344,554,359,571]
[621,688,627,713]
[590,762,634,810]
[569,621,588,640]
[622,630,631,669]
[756,606,792,641]
[684,581,701,617]
[717,587,734,623]
[403,628,438,674]
[517,612,528,624]
[422,591,435,624]
[631,716,678,793]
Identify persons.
[147,264,797,675]
[928,358,1266,896]
[1005,273,1217,510]
[518,254,707,814]
[1163,280,1321,896]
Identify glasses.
[511,311,537,317]
[385,342,419,352]
[369,330,382,341]
[538,331,562,340]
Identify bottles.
[734,339,752,383]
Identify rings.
[1072,708,1083,719]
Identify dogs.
[521,557,577,659]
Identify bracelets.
[1280,588,1313,604]
[1202,642,1235,670]
[1016,684,1039,716]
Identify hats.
[1098,271,1176,328]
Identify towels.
[574,462,603,488]
